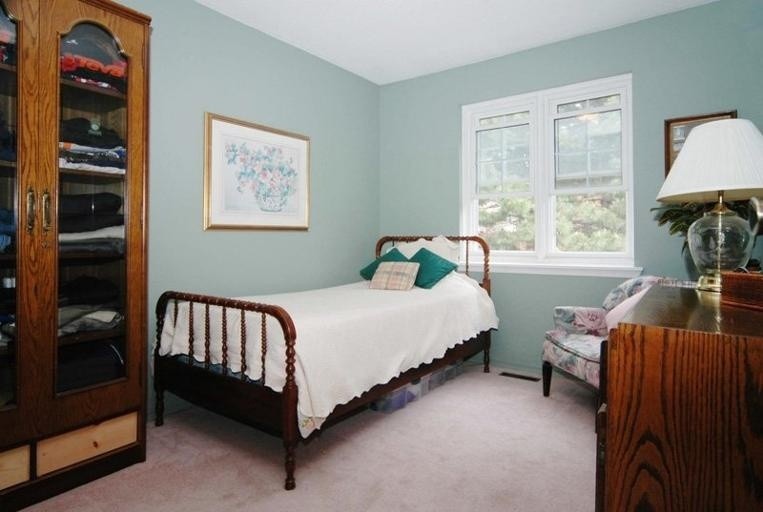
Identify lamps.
[654,116,763,215]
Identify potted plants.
[649,198,757,282]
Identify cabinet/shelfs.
[0,0,152,512]
[595,285,763,511]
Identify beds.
[154,234,492,492]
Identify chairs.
[539,276,664,411]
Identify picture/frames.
[202,109,312,232]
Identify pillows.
[358,234,461,291]
